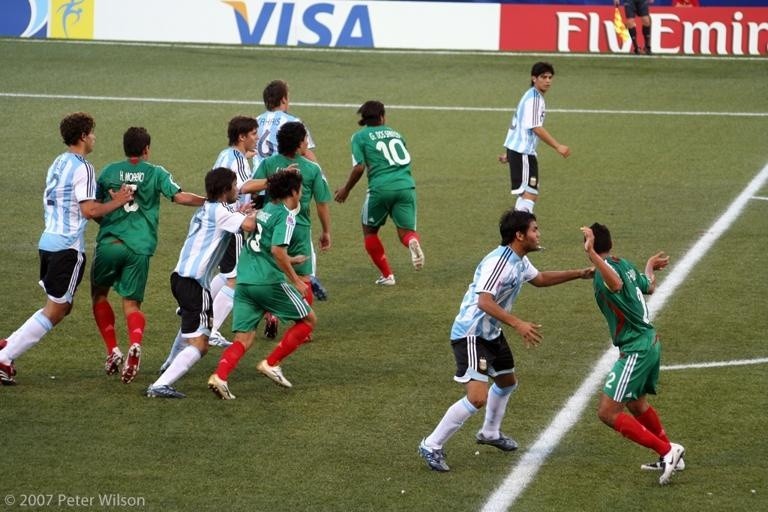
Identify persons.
[579,222,686,486]
[499,62,572,251]
[0,113,135,386]
[146,80,425,399]
[417,207,597,472]
[614,0,654,56]
[90,126,207,385]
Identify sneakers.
[418,436,450,472]
[656,443,685,486]
[474,429,519,451]
[640,457,685,471]
